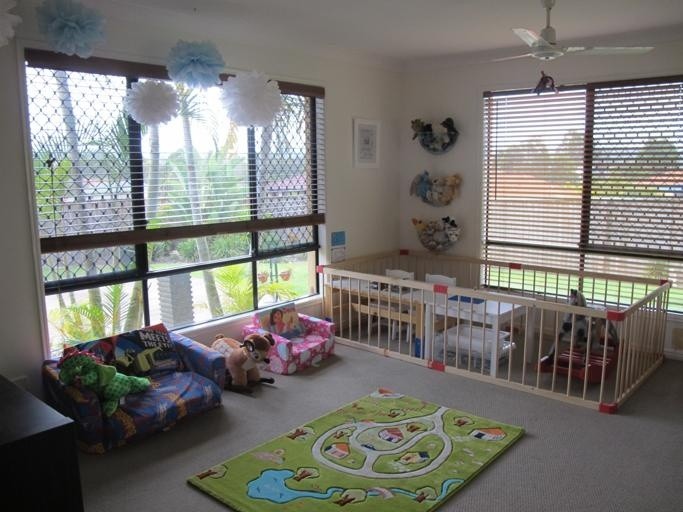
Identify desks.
[333,276,535,377]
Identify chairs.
[365,268,457,344]
[243,312,336,376]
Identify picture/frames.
[354,118,380,169]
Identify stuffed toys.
[56,348,152,417]
[408,117,460,254]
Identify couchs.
[42,331,227,457]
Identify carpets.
[186,386,524,512]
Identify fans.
[477,0,656,64]
